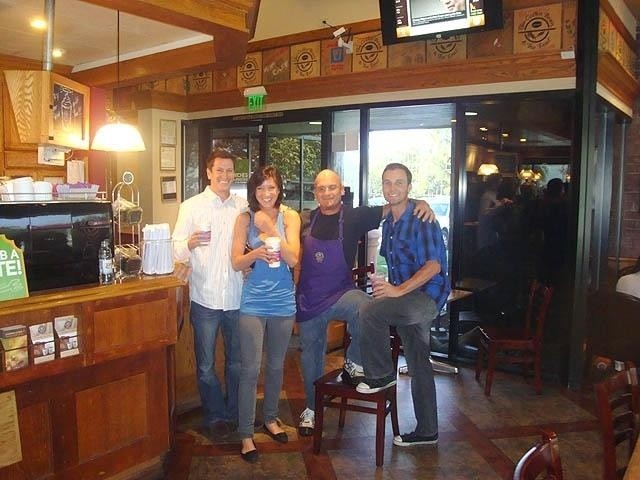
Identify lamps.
[517,138,535,180]
[531,140,543,180]
[478,124,499,176]
[89,10,147,153]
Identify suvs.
[227,181,319,216]
[419,195,450,249]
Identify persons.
[230,165,301,463]
[474,172,571,325]
[294,168,437,436]
[354,162,451,447]
[170,149,263,440]
[440,0,466,13]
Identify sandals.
[263,420,288,443]
[240,433,258,462]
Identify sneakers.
[299,404,328,436]
[208,417,249,439]
[393,428,439,447]
[356,371,399,394]
[342,360,366,385]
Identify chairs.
[473,277,554,400]
[585,290,639,390]
[593,359,640,479]
[513,428,563,479]
[312,315,403,470]
[343,262,402,373]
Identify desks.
[397,287,476,376]
[453,275,502,346]
[621,428,640,479]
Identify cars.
[369,196,389,227]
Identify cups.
[264,237,282,269]
[196,220,211,246]
[1,176,53,201]
[370,272,385,300]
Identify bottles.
[98,241,115,285]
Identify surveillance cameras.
[332,26,346,37]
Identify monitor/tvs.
[377,0,504,47]
